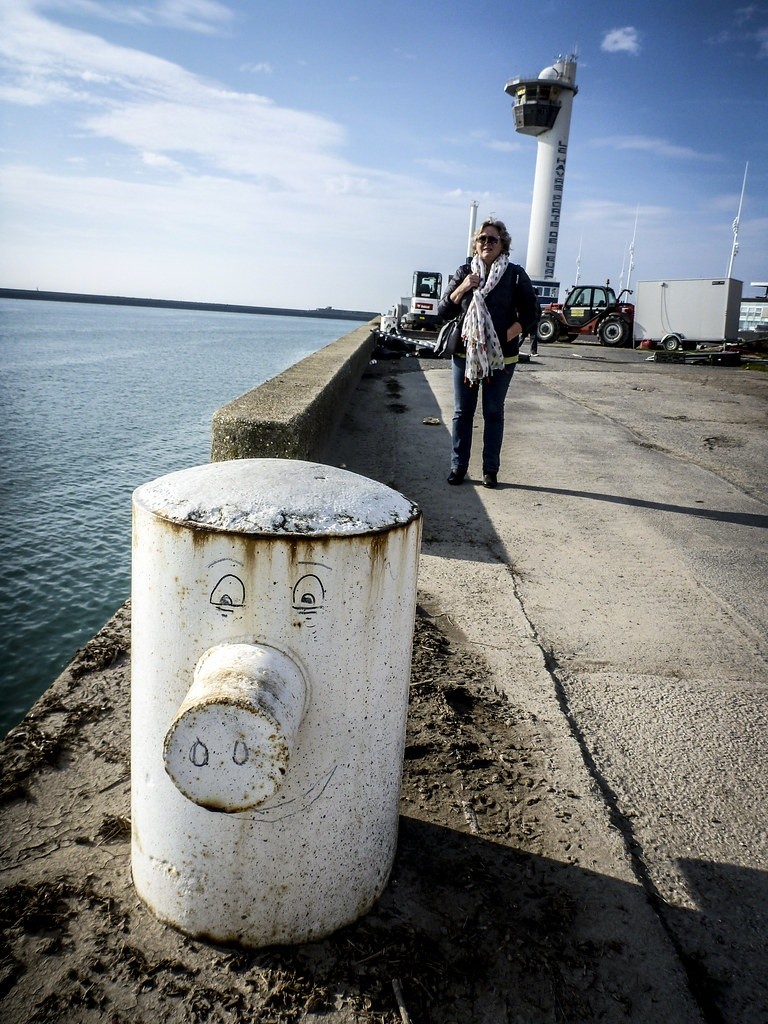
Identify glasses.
[477,234,500,245]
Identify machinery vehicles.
[536,278,635,349]
[400,269,443,332]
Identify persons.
[518,287,542,356]
[435,221,543,489]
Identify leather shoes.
[447,467,466,484]
[482,469,498,488]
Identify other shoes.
[531,352,540,357]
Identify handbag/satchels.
[433,316,459,357]
